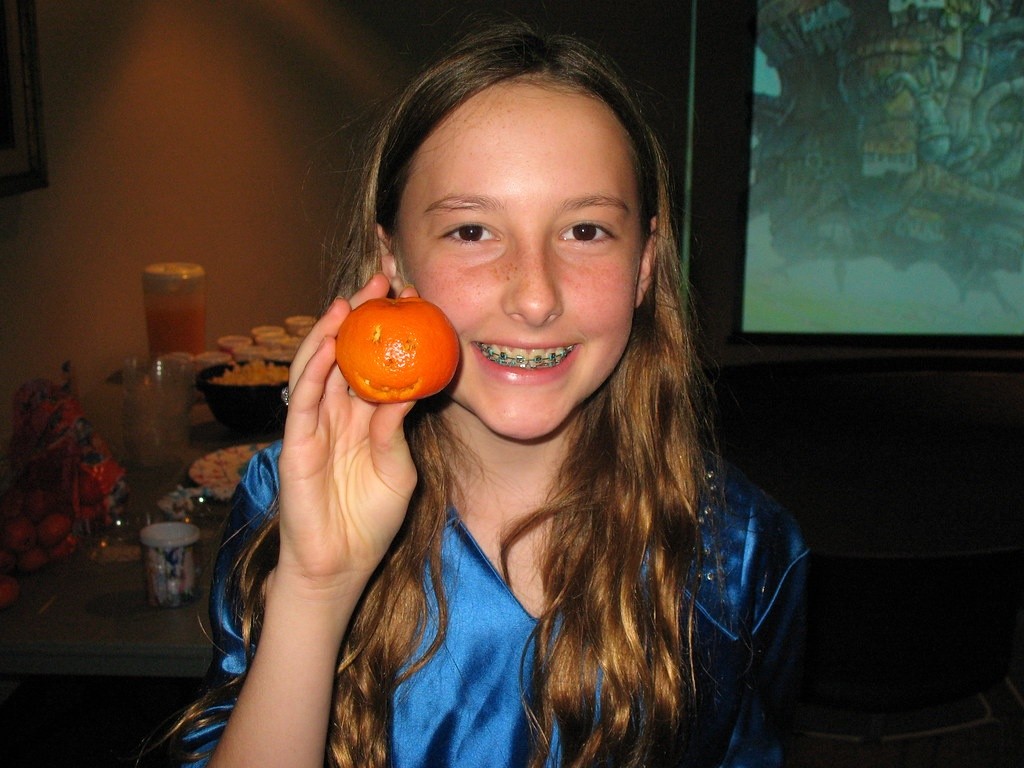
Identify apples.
[0,504,79,609]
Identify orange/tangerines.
[334,298,461,402]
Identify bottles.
[125,362,193,467]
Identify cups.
[139,521,211,610]
[143,261,206,362]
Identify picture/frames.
[0,0,50,197]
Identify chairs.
[791,547,1024,768]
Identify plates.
[188,444,277,503]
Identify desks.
[0,490,227,715]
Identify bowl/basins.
[198,359,291,434]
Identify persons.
[151,27,808,768]
